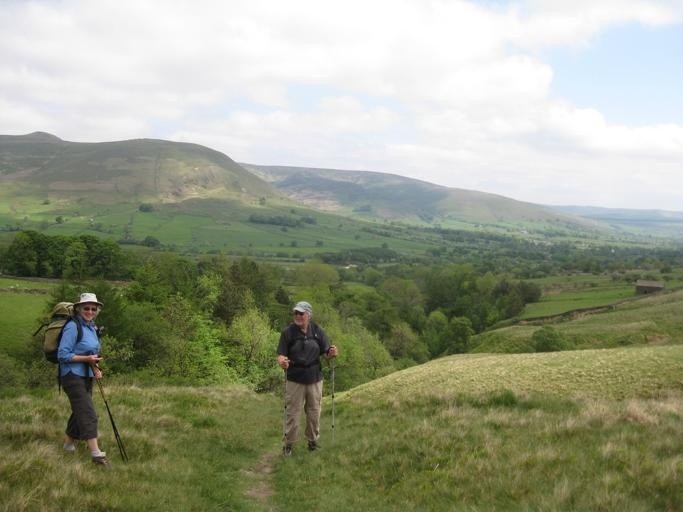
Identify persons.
[275,300,338,457]
[56,293,109,469]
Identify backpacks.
[43,302,83,364]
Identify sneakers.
[308,441,320,451]
[92,456,113,472]
[283,444,293,457]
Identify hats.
[73,293,105,310]
[293,301,312,314]
